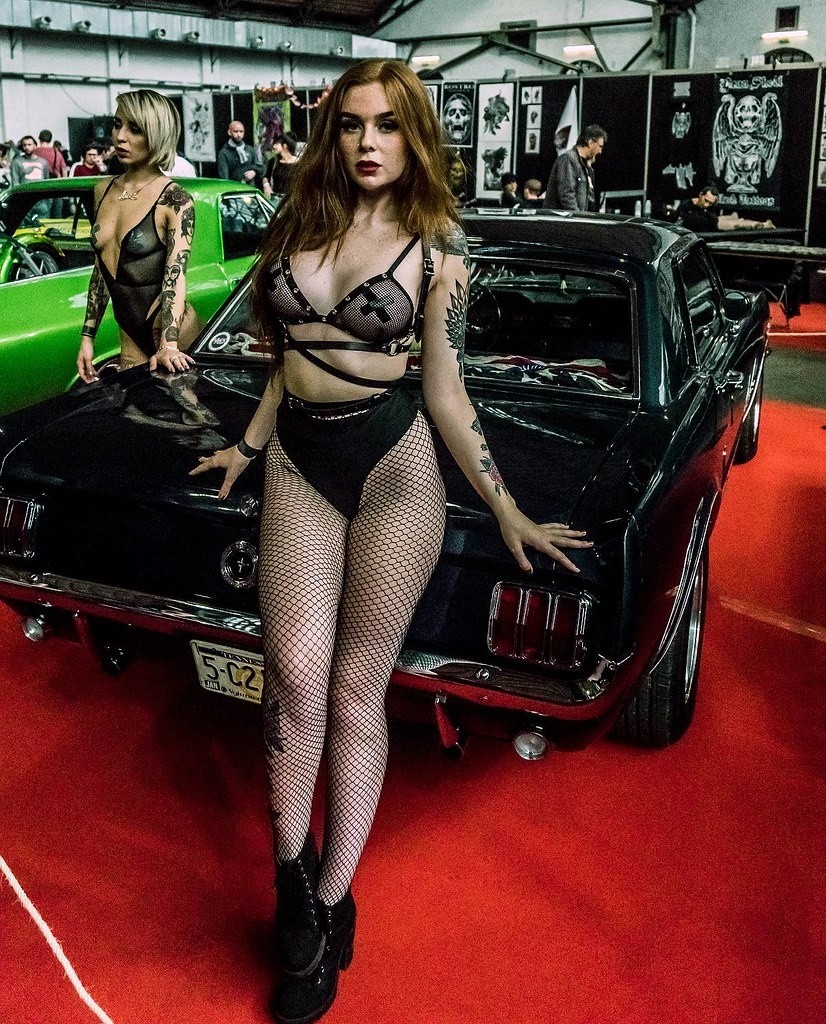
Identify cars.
[3,200,773,762]
[0,177,286,418]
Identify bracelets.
[263,181,270,188]
[155,345,180,353]
[236,437,262,461]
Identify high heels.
[275,828,326,974]
[271,886,357,1023]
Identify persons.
[674,180,719,229]
[0,126,130,228]
[187,59,600,1024]
[667,207,777,230]
[216,121,264,222]
[75,89,205,383]
[259,132,308,200]
[517,179,545,210]
[158,146,197,179]
[541,123,608,215]
[500,172,521,208]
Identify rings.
[170,356,179,362]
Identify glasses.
[593,138,604,149]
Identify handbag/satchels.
[49,172,57,178]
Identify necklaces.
[119,172,163,203]
